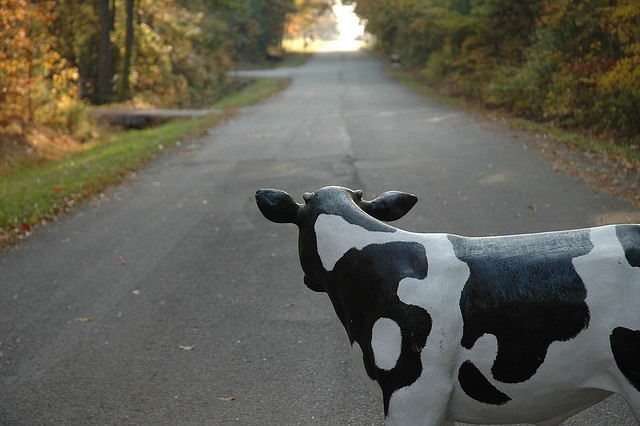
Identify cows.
[253,184,640,426]
